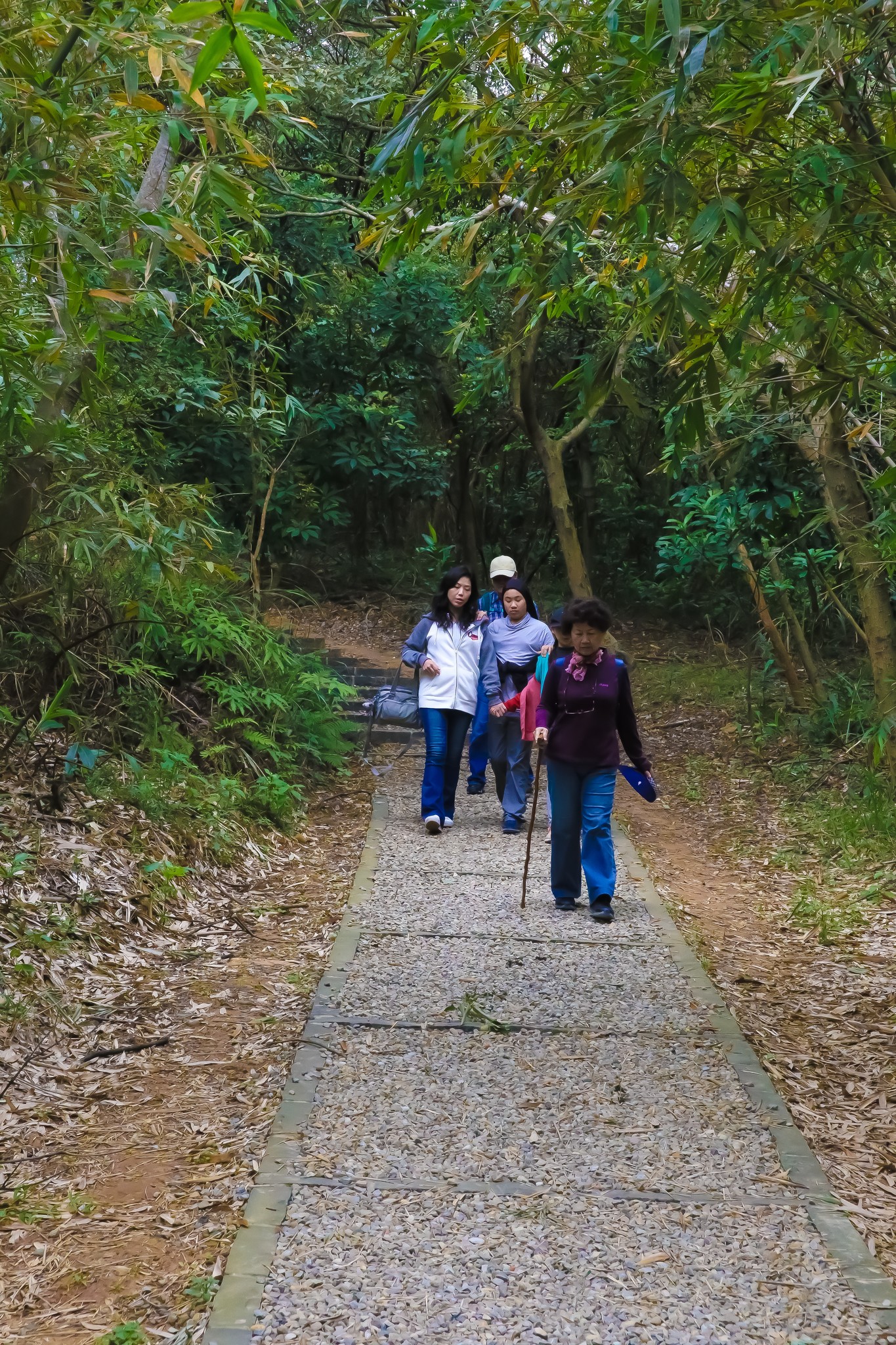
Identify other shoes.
[544,828,551,841]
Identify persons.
[401,566,507,834]
[475,580,555,832]
[466,555,533,795]
[490,607,575,843]
[534,597,653,919]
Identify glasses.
[563,684,594,715]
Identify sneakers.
[554,897,576,909]
[466,784,485,794]
[444,817,453,827]
[526,784,534,794]
[503,820,520,832]
[590,899,615,920]
[424,815,442,832]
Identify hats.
[550,608,566,626]
[617,763,660,803]
[490,555,516,578]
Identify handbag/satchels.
[373,658,424,730]
